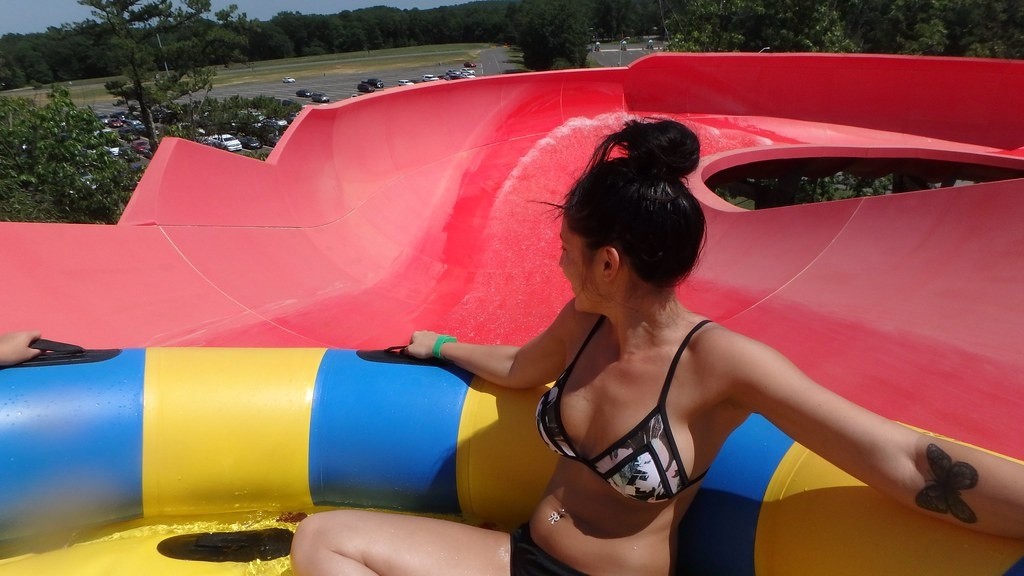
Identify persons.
[290,120,1024,576]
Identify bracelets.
[432,335,457,359]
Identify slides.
[0,50,1024,471]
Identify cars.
[281,61,476,103]
[97,105,299,159]
